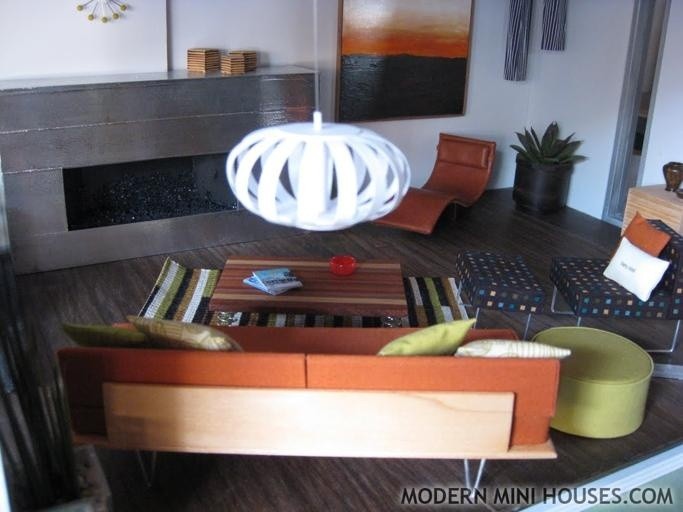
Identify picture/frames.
[334,0,475,126]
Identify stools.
[528,326,653,441]
[453,248,545,340]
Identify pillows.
[57,319,155,349]
[123,314,244,353]
[609,211,672,259]
[373,317,477,358]
[451,337,572,360]
[600,236,671,302]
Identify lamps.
[223,0,414,232]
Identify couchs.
[54,320,563,504]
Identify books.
[243,276,288,296]
[252,267,304,292]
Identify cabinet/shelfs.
[618,183,682,237]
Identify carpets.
[135,254,469,326]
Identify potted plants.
[508,120,589,215]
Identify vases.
[661,161,682,193]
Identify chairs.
[366,132,497,247]
[546,218,681,355]
